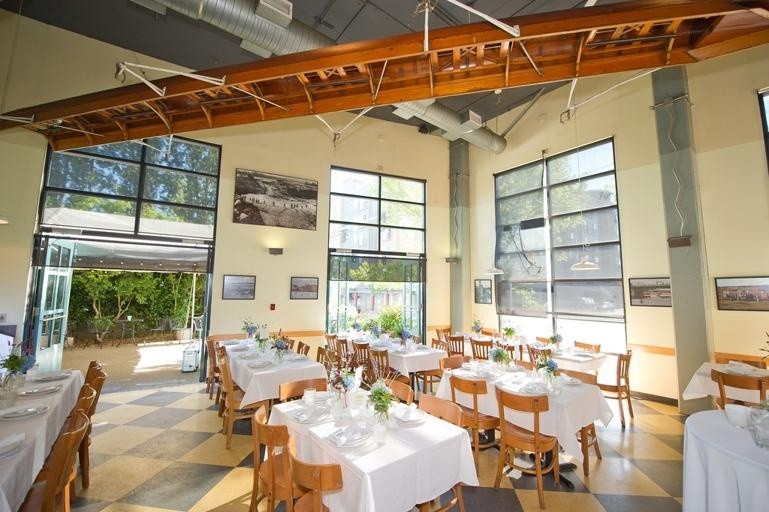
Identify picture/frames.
[290,277,319,299]
[474,279,492,304]
[222,275,255,301]
[715,275,769,311]
[629,277,672,307]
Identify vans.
[577,298,596,311]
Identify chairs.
[64,315,202,351]
[0,360,107,512]
[713,352,769,370]
[710,369,769,410]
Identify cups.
[722,401,769,449]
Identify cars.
[598,301,615,312]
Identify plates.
[338,332,430,355]
[222,338,310,371]
[290,385,426,451]
[459,356,583,399]
[0,370,70,460]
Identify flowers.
[375,308,404,333]
[356,319,373,329]
[472,314,482,331]
[0,338,36,387]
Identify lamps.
[570,91,600,272]
